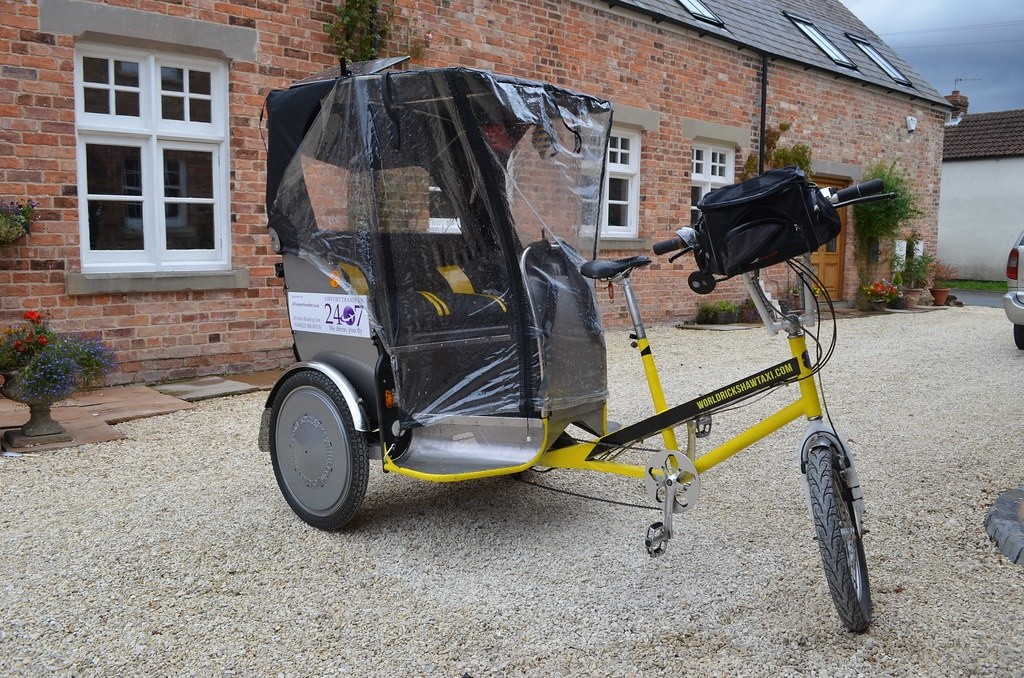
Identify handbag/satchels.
[698,166,843,277]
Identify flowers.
[0,306,124,410]
[813,283,824,295]
[861,281,899,303]
[0,196,42,244]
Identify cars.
[1003,231,1024,350]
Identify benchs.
[292,236,528,345]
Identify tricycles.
[256,55,900,634]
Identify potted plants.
[695,298,738,324]
[928,257,960,306]
[884,250,940,307]
[790,286,801,310]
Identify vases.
[1,373,64,436]
[870,299,887,312]
[815,294,822,303]
